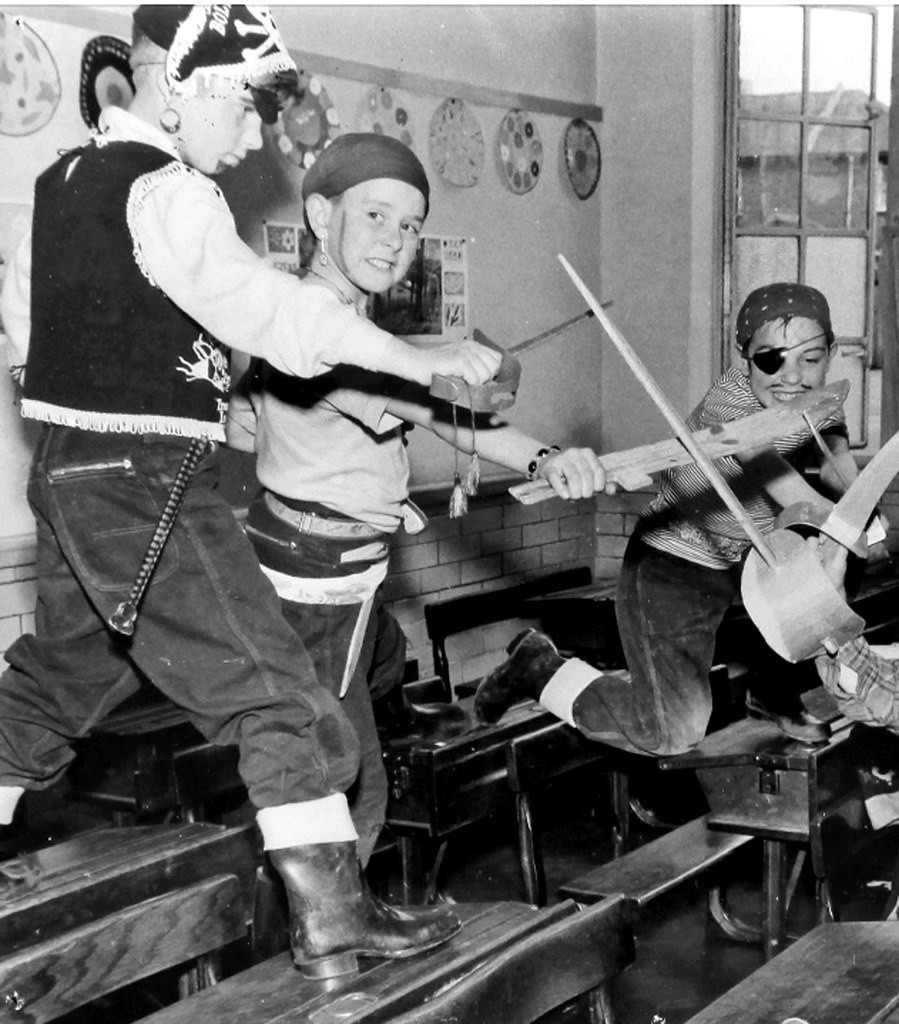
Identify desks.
[0,583,899,1024]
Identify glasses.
[244,81,285,124]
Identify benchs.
[384,892,640,1024]
[425,566,593,704]
[807,726,899,921]
[0,875,248,1024]
[508,722,754,1024]
[174,676,444,905]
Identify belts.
[262,492,385,541]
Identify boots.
[368,681,466,744]
[254,791,464,981]
[742,680,833,743]
[471,627,604,729]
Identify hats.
[134,5,300,93]
[301,132,430,220]
[734,281,833,345]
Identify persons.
[809,538,899,924]
[0,4,502,980]
[472,281,889,757]
[234,132,609,869]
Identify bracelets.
[526,446,562,480]
